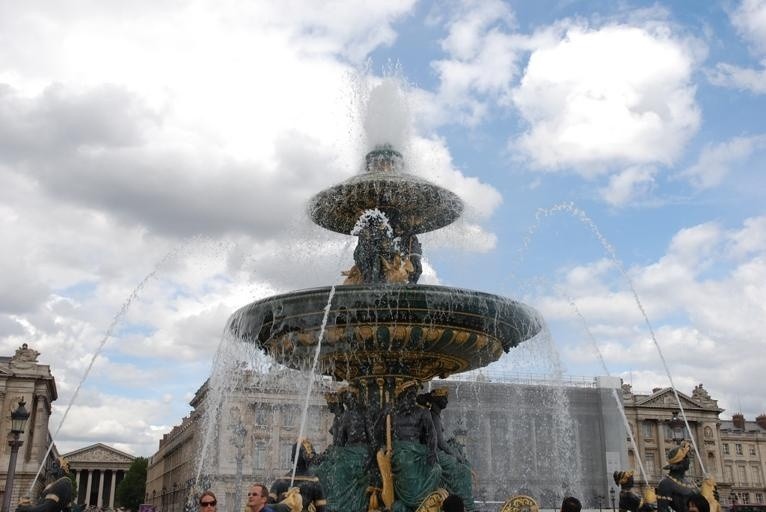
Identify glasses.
[248,493,258,496]
[200,501,216,506]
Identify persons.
[248,483,276,512]
[317,380,475,512]
[197,491,217,512]
[561,497,582,512]
[612,446,720,512]
